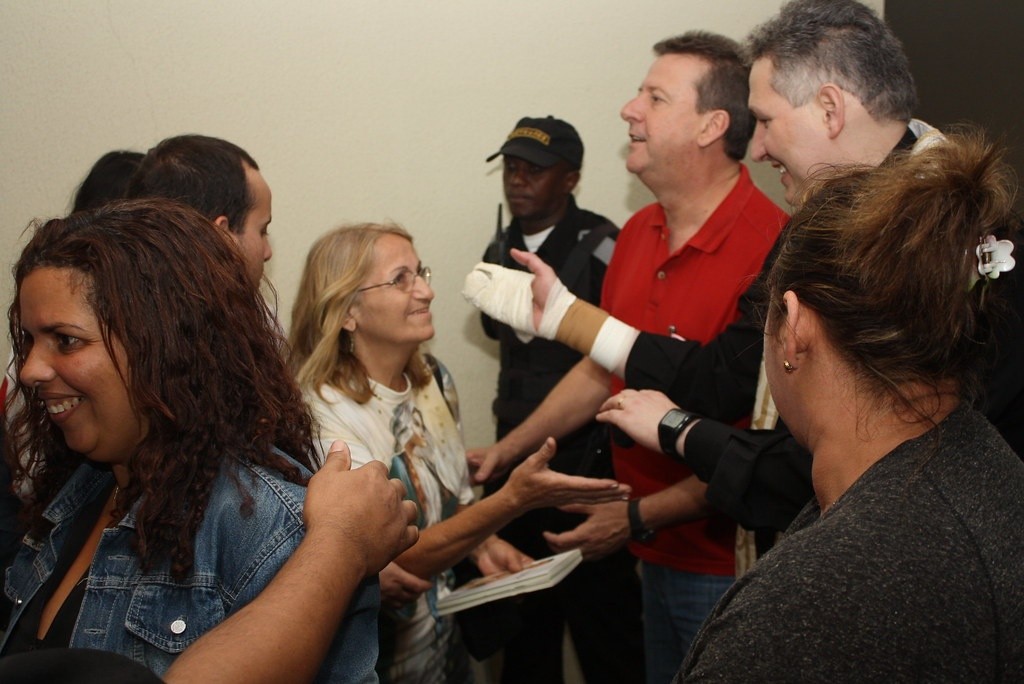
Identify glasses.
[356,266,431,293]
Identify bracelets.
[628,501,655,543]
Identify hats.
[486,115,583,169]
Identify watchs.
[659,409,701,459]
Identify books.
[436,548,584,616]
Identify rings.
[618,396,626,408]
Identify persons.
[465,32,791,684]
[0,196,419,684]
[129,135,273,293]
[288,222,632,684]
[462,0,1024,532]
[669,124,1024,684]
[73,153,145,213]
[479,117,648,684]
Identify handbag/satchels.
[454,559,502,663]
